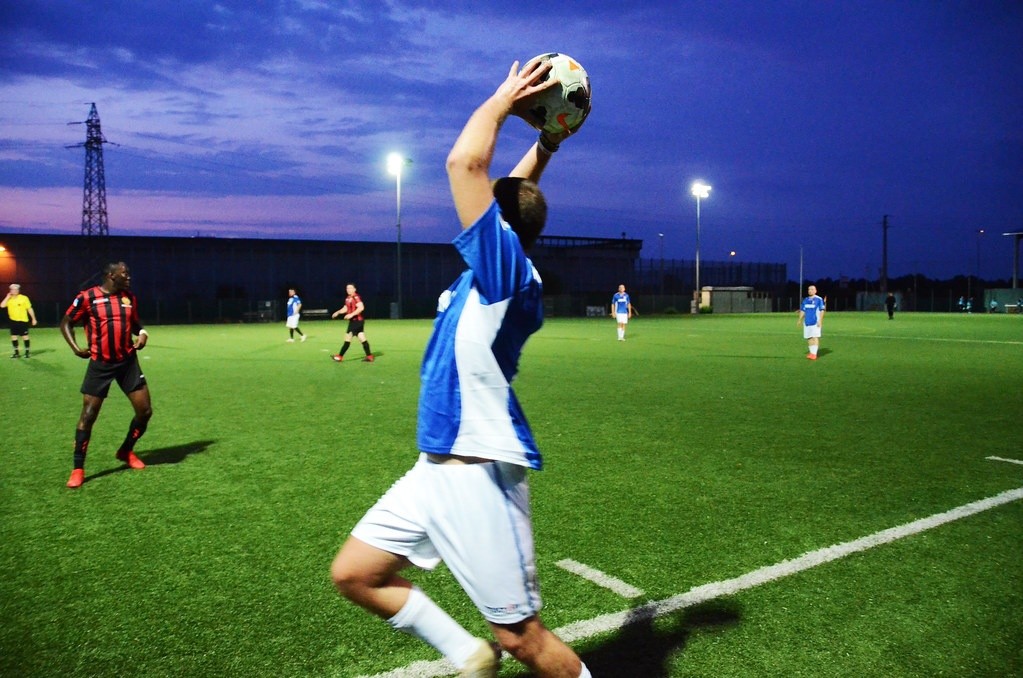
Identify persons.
[611,284,631,342]
[285,289,306,343]
[59,262,153,487]
[884,292,896,320]
[332,281,374,362]
[797,286,826,360]
[958,296,1023,314]
[0,284,37,358]
[329,58,592,678]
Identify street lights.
[389,154,406,320]
[689,178,712,310]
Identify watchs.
[540,132,559,153]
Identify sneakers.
[66,469,85,487]
[117,449,145,469]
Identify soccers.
[517,53,592,134]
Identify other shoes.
[301,335,306,342]
[807,353,817,360]
[362,354,373,363]
[330,354,343,362]
[286,338,294,342]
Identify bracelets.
[537,138,553,158]
[139,329,149,338]
